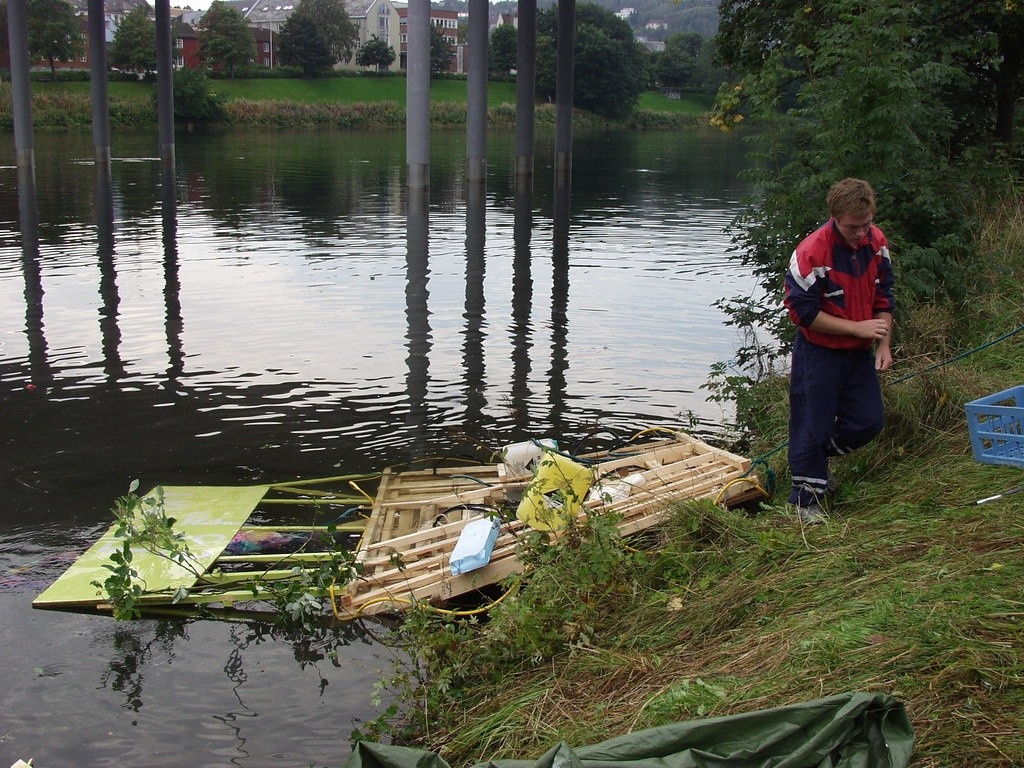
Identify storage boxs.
[963,386,1024,468]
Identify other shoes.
[798,504,825,524]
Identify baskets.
[964,385,1024,468]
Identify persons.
[783,178,895,523]
